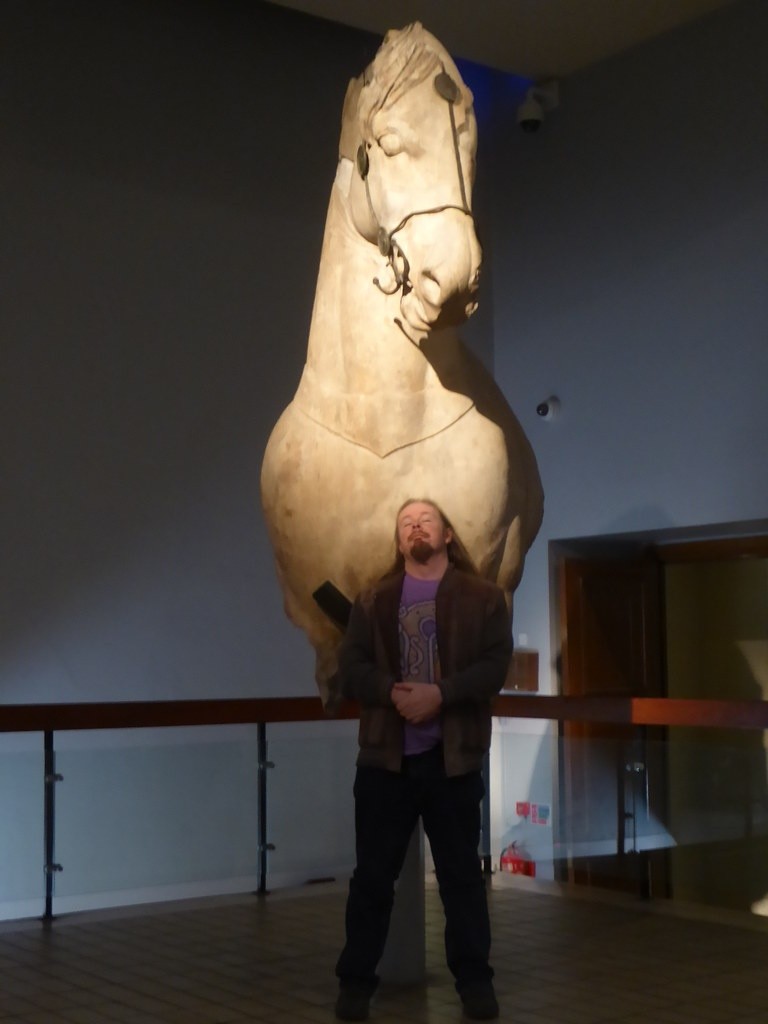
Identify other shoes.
[460,979,499,1019]
[335,975,374,1019]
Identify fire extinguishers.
[499,840,523,873]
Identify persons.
[336,498,514,1021]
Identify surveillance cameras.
[535,394,560,422]
[515,96,546,134]
